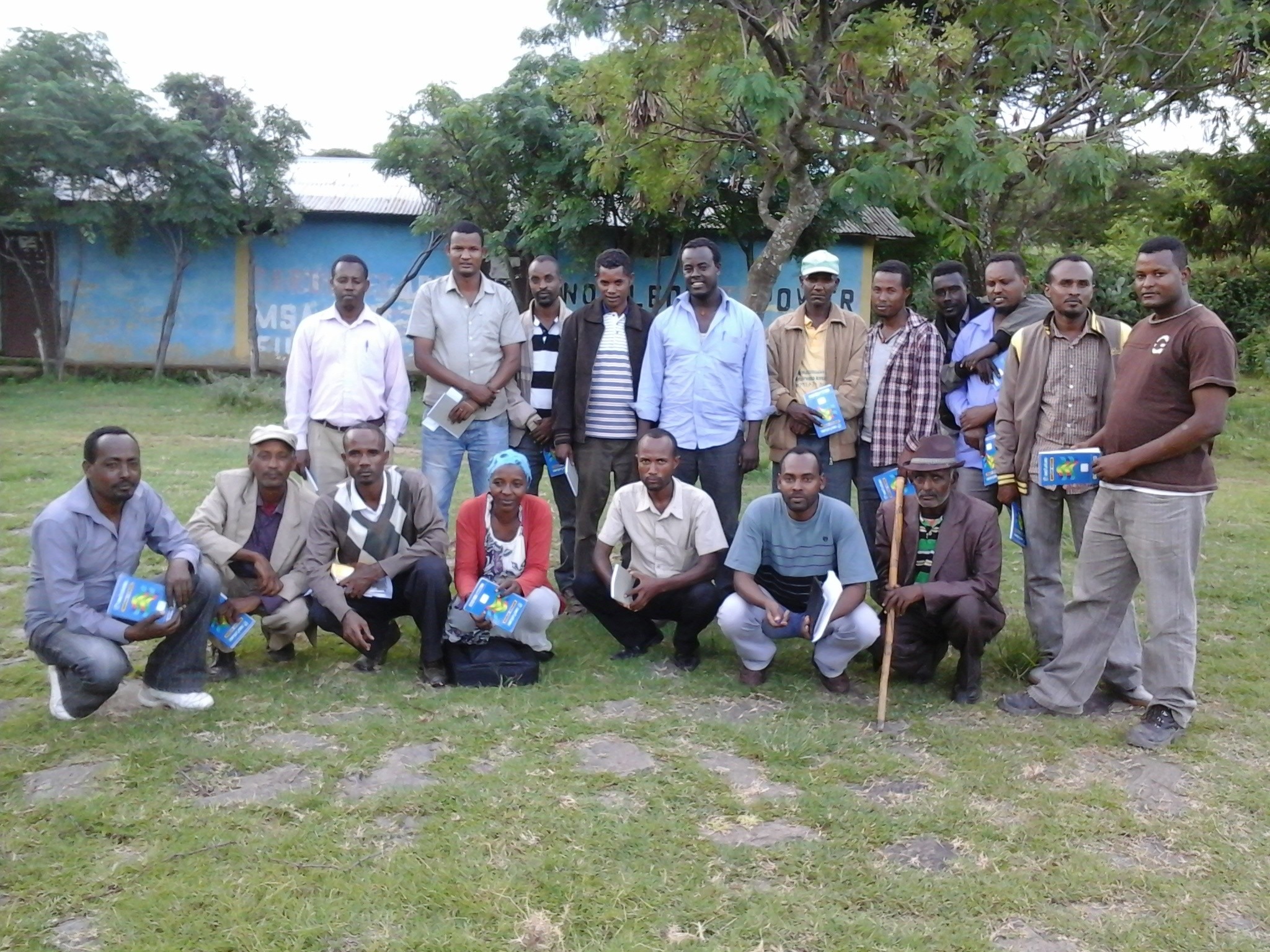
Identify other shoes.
[956,668,981,703]
[208,649,235,681]
[423,660,449,686]
[811,652,850,693]
[741,666,766,687]
[1027,665,1047,685]
[354,621,402,671]
[613,629,665,662]
[673,636,701,672]
[267,643,296,662]
[1118,684,1153,706]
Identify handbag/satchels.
[447,637,539,685]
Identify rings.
[459,408,463,411]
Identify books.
[463,576,528,633]
[805,570,843,644]
[803,384,847,438]
[610,564,636,605]
[982,431,998,487]
[543,446,565,477]
[421,387,476,439]
[106,572,176,625]
[1038,446,1103,486]
[872,467,916,501]
[564,457,579,497]
[331,563,392,599]
[1009,501,1026,548]
[209,592,256,649]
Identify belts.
[317,416,387,432]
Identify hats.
[897,435,965,473]
[801,249,840,277]
[249,423,298,452]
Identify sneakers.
[138,684,215,709]
[48,665,73,720]
[1128,706,1183,749]
[996,690,1052,717]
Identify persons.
[23,426,222,721]
[183,425,321,683]
[304,421,453,689]
[285,254,411,498]
[405,219,1236,751]
